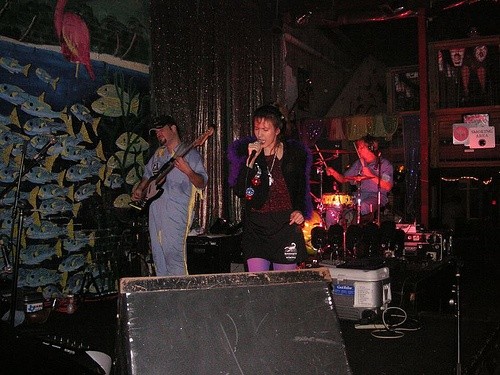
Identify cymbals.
[308,180,323,184]
[343,174,376,181]
[314,149,357,154]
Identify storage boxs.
[329,267,390,321]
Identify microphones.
[33,135,57,159]
[250,140,261,159]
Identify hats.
[148,114,178,137]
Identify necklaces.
[267,142,279,186]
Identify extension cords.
[354,323,390,329]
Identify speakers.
[112,266,354,375]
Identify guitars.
[131,127,214,210]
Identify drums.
[322,192,353,209]
[301,209,329,255]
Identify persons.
[325,134,393,216]
[227,105,313,272]
[131,113,209,277]
